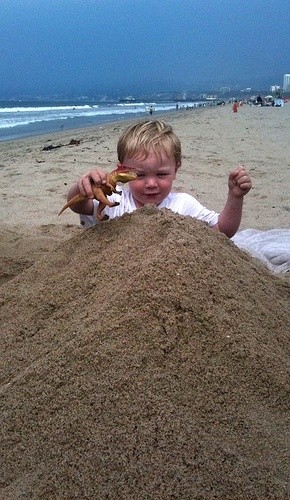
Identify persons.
[232,100,238,112]
[66,120,253,239]
[149,106,153,116]
